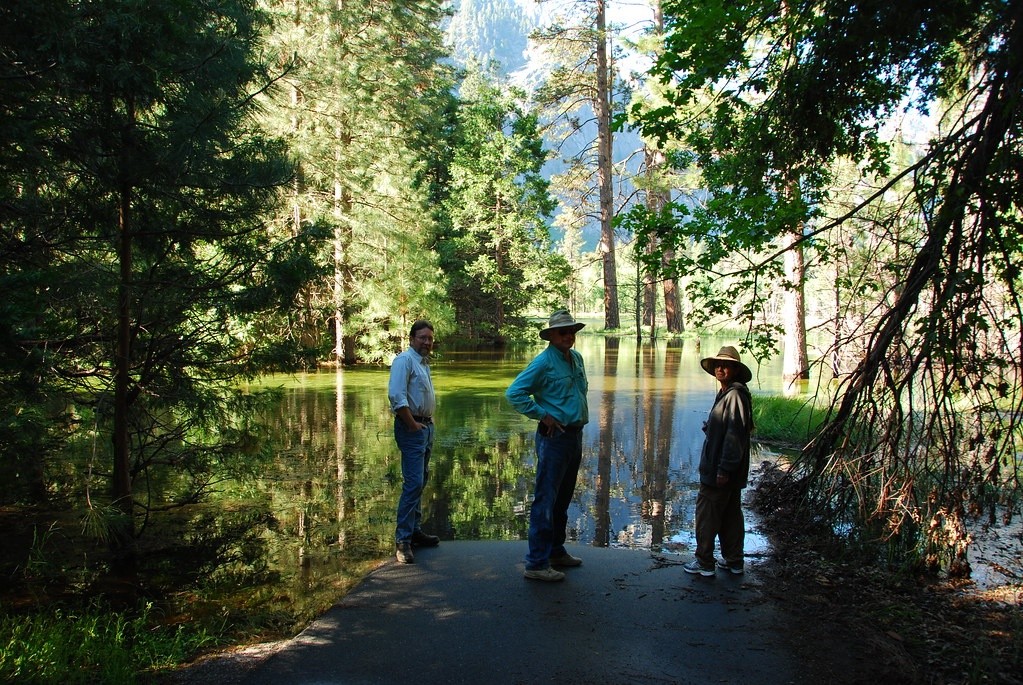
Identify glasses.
[553,330,575,336]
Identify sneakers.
[683,558,716,577]
[403,531,439,547]
[550,550,583,566]
[522,566,564,581]
[715,552,745,573]
[396,542,413,562]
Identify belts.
[561,427,584,433]
[396,413,432,422]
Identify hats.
[540,308,585,339]
[701,346,752,384]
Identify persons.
[505,309,588,581]
[388,320,440,563]
[684,345,753,577]
